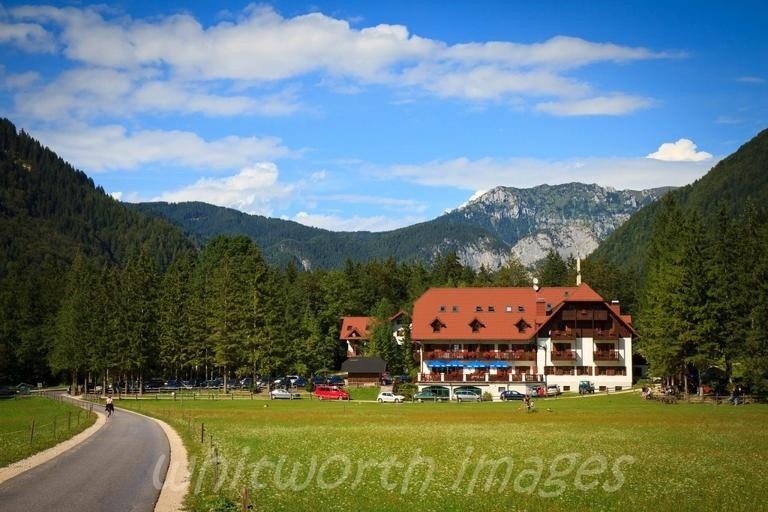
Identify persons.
[530,400,534,408]
[732,388,739,406]
[641,385,647,397]
[665,386,671,394]
[104,394,113,412]
[643,386,650,399]
[669,386,675,396]
[524,395,530,413]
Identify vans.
[452,391,482,402]
[314,384,350,400]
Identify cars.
[79,374,346,395]
[413,392,450,403]
[268,389,301,400]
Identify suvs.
[547,383,562,395]
[577,380,595,393]
[375,391,405,403]
[530,386,543,397]
[500,390,530,400]
